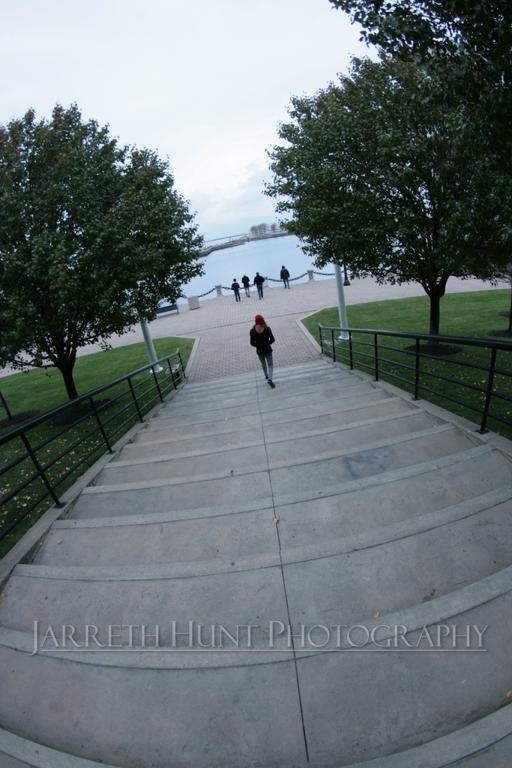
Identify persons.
[230,271,265,302]
[249,315,277,389]
[280,265,291,289]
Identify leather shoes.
[265,376,275,388]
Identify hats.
[255,315,265,325]
